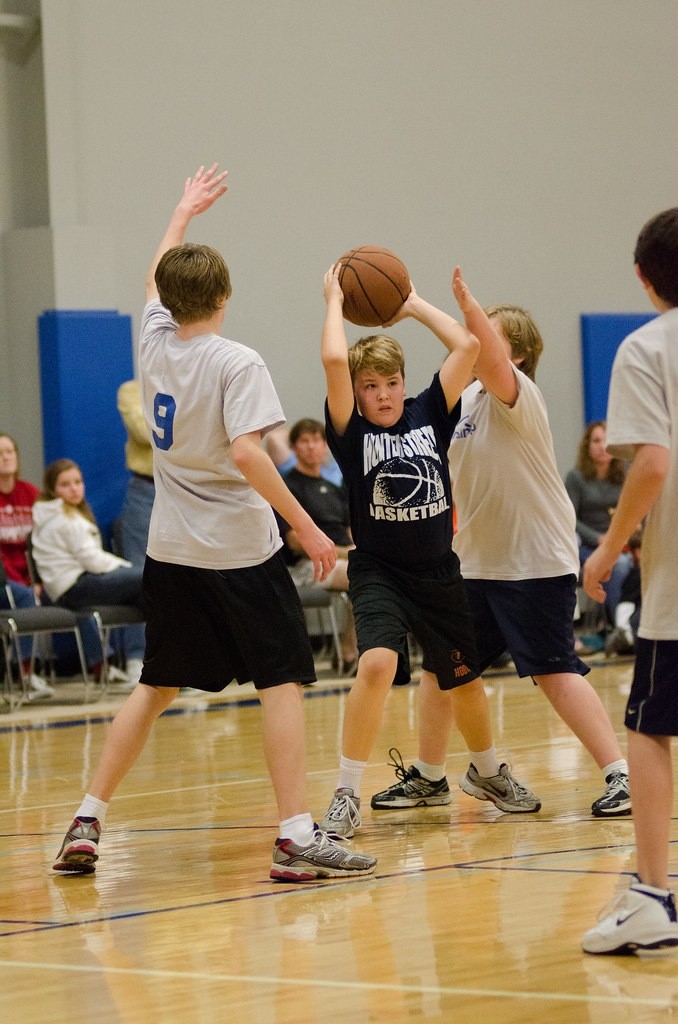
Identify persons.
[0,380,355,702]
[565,206,678,954]
[53,158,381,884]
[321,262,631,840]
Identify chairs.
[0,518,602,713]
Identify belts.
[133,471,154,484]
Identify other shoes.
[573,643,598,655]
[125,660,144,683]
[95,663,131,682]
[332,653,361,677]
[18,673,55,698]
[597,619,612,639]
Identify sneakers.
[269,821,378,880]
[370,764,453,810]
[592,773,632,817]
[460,762,541,814]
[54,873,117,953]
[323,787,361,839]
[53,816,101,873]
[583,955,678,1009]
[582,874,678,955]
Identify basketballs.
[331,247,411,327]
